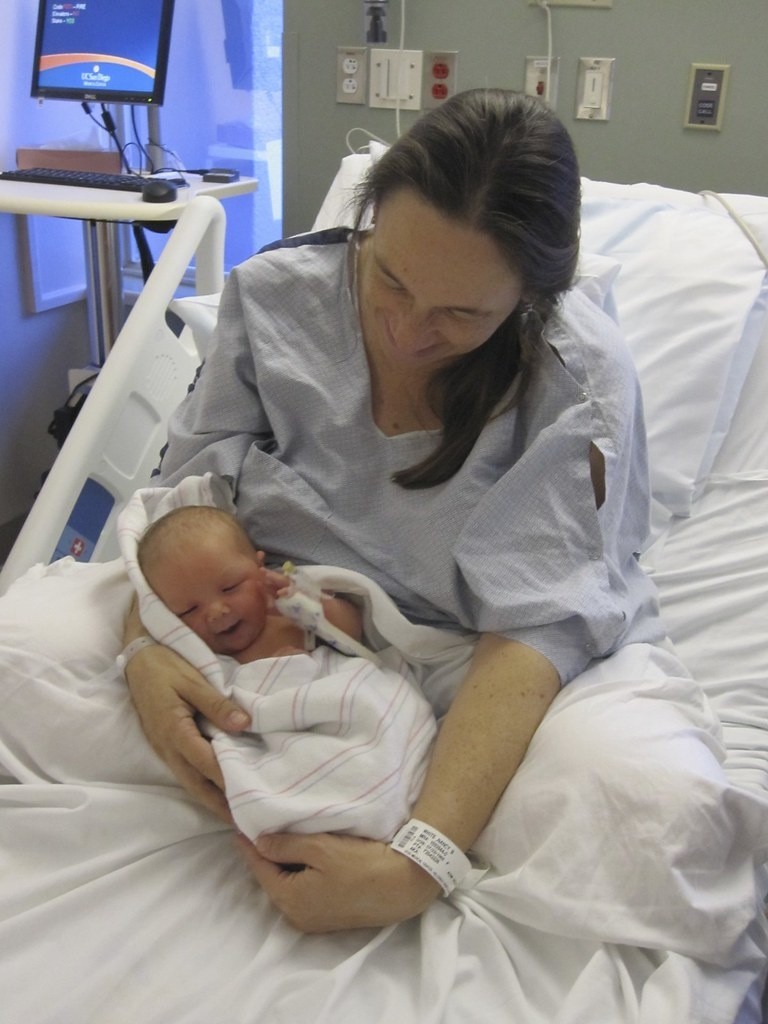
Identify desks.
[0,172,261,413]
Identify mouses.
[142,181,177,204]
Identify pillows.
[368,138,768,519]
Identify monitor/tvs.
[31,0,175,107]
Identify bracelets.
[115,635,155,684]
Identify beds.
[0,146,768,1024]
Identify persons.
[114,88,648,937]
[138,506,439,840]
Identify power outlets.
[423,51,457,109]
[337,46,368,105]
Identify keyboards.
[0,167,186,193]
